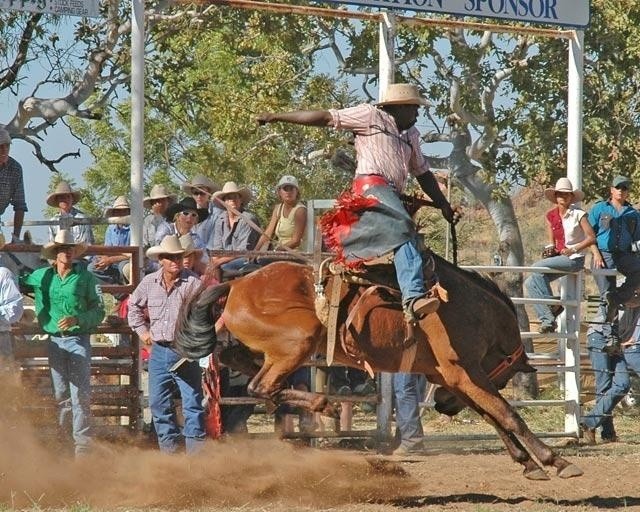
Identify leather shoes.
[537,325,555,335]
[403,296,441,325]
[577,421,598,443]
[550,306,564,318]
[600,430,620,442]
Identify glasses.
[193,191,205,195]
[180,211,198,217]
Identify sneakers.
[601,339,621,355]
[604,290,615,314]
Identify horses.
[0,230,52,292]
[160,249,584,481]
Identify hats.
[108,195,131,210]
[0,128,13,146]
[544,177,586,204]
[39,227,88,261]
[45,181,81,207]
[212,181,251,207]
[373,82,428,107]
[144,232,187,264]
[611,175,634,188]
[166,196,210,221]
[277,175,299,189]
[179,231,205,255]
[142,183,178,208]
[118,257,132,283]
[181,174,217,192]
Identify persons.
[46,181,95,245]
[330,366,375,396]
[19,229,105,460]
[619,305,640,379]
[182,174,216,209]
[148,197,210,272]
[127,234,205,456]
[86,196,131,314]
[142,184,177,247]
[587,175,640,354]
[212,181,261,251]
[579,306,632,446]
[0,130,28,243]
[254,83,463,323]
[385,373,427,455]
[220,175,307,275]
[0,267,24,360]
[524,177,596,333]
